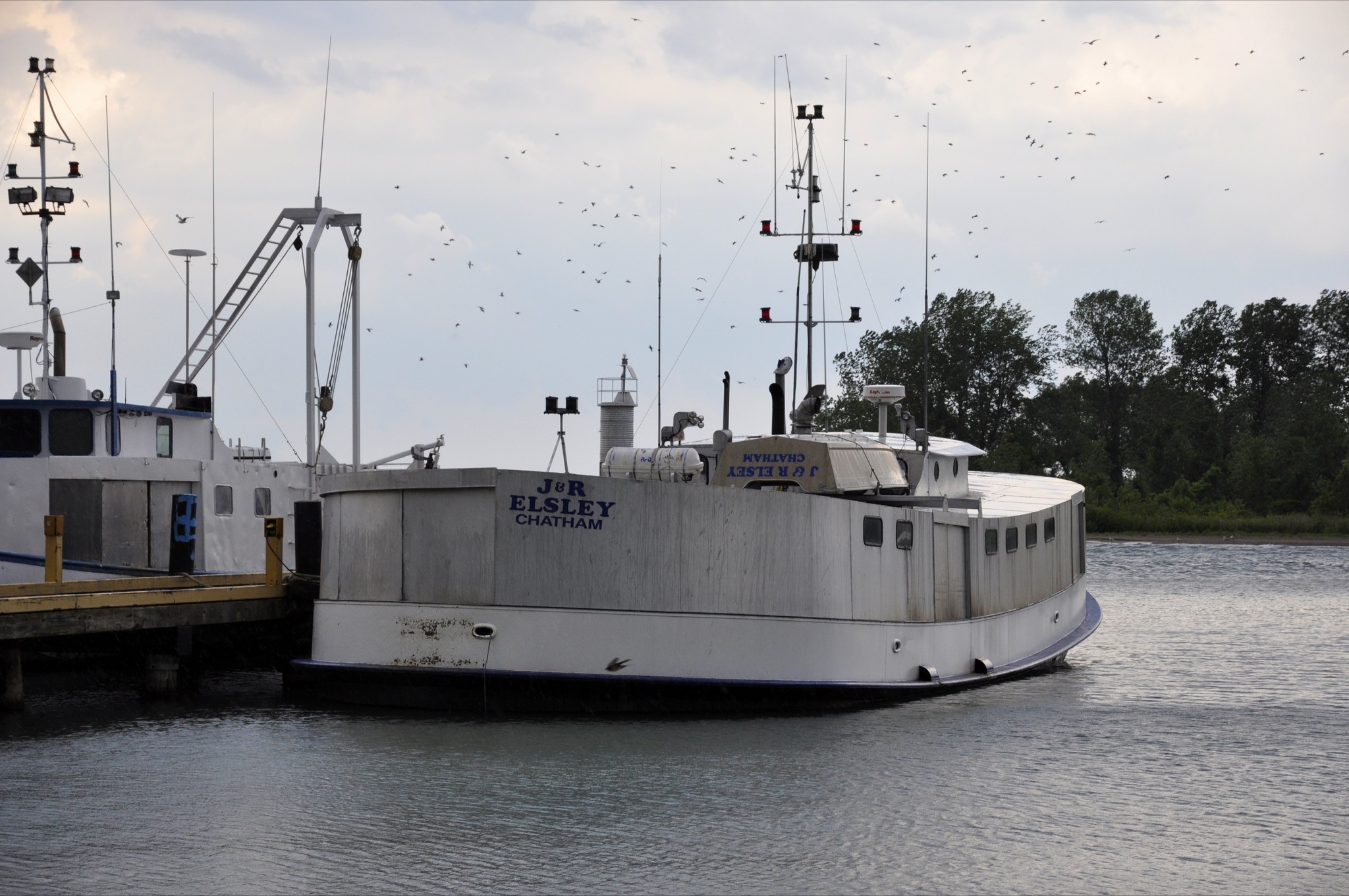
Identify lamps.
[91,390,103,401]
[901,411,910,421]
[23,383,39,400]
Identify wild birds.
[83,32,1349,385]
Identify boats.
[293,54,1104,719]
[1,54,445,577]
